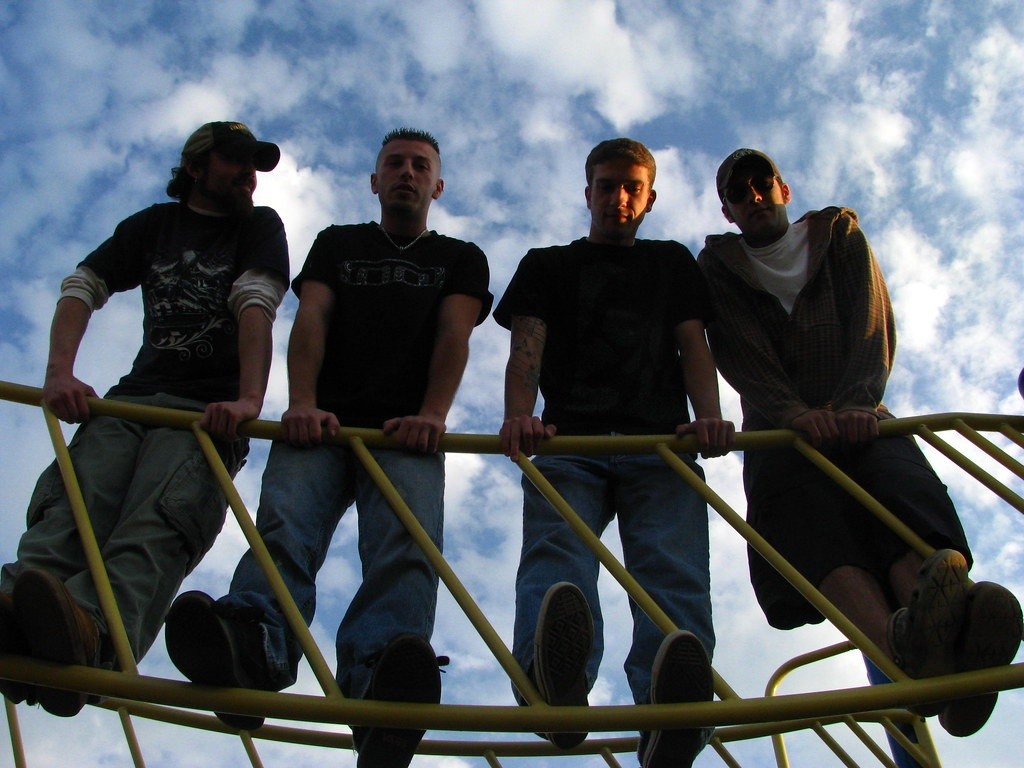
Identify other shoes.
[357,633,449,768]
[636,630,714,768]
[0,569,99,717]
[536,582,592,749]
[165,588,265,731]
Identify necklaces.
[375,220,430,253]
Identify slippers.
[937,581,1024,737]
[887,549,970,717]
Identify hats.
[717,149,783,205]
[182,121,281,172]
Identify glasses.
[721,173,774,206]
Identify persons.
[695,148,1023,735]
[493,139,735,768]
[0,120,293,718]
[164,132,495,768]
